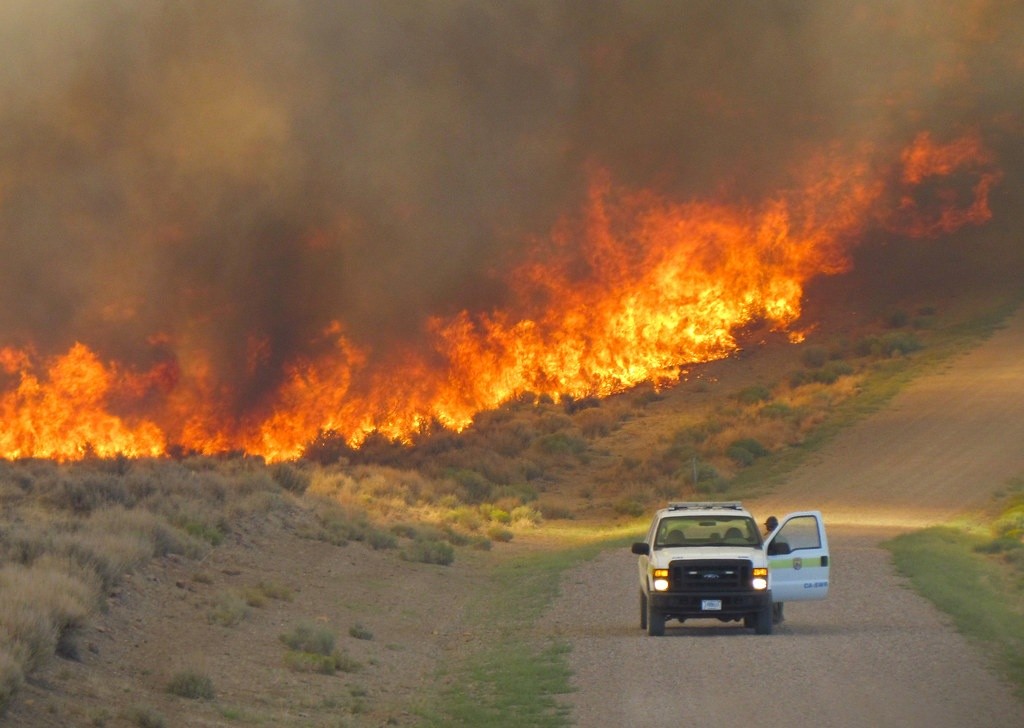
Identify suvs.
[629,501,832,637]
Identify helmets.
[764,516,778,527]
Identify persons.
[763,517,787,621]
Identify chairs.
[664,529,684,545]
[722,528,743,544]
